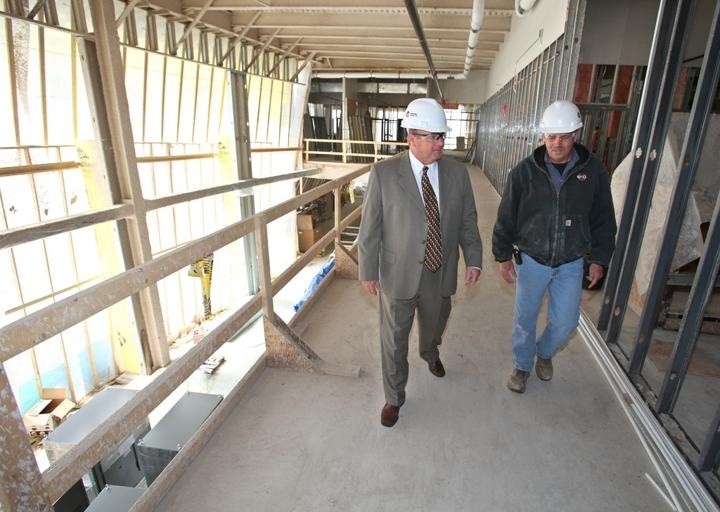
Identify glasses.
[412,133,446,140]
[543,133,576,142]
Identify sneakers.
[535,356,553,381]
[507,368,530,393]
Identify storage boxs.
[296,213,325,252]
[24,388,77,431]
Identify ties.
[422,166,442,274]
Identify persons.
[490,99,617,394]
[356,97,484,427]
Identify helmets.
[400,98,452,133]
[539,100,584,133]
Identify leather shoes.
[429,357,445,377]
[381,403,400,426]
